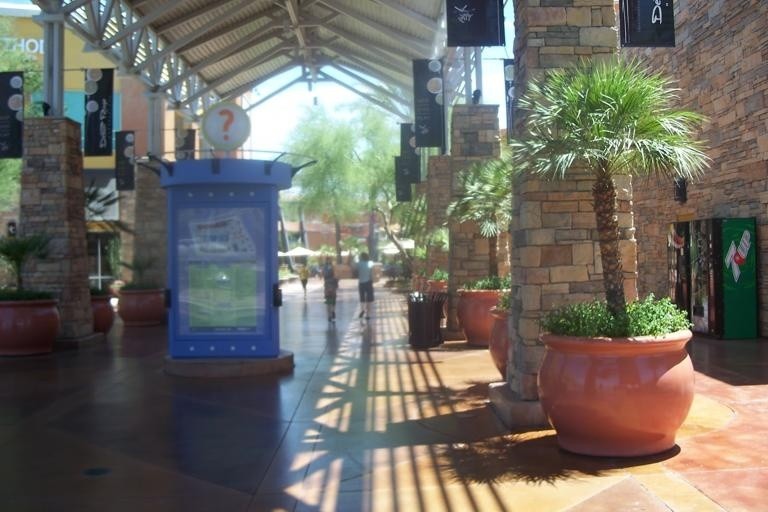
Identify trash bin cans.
[403,291,448,348]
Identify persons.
[349,251,375,320]
[298,263,309,293]
[321,256,338,322]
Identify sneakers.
[359,310,370,319]
[328,311,335,322]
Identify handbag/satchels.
[371,268,379,282]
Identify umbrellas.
[285,247,315,256]
[278,250,286,256]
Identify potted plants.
[0,230,168,357]
